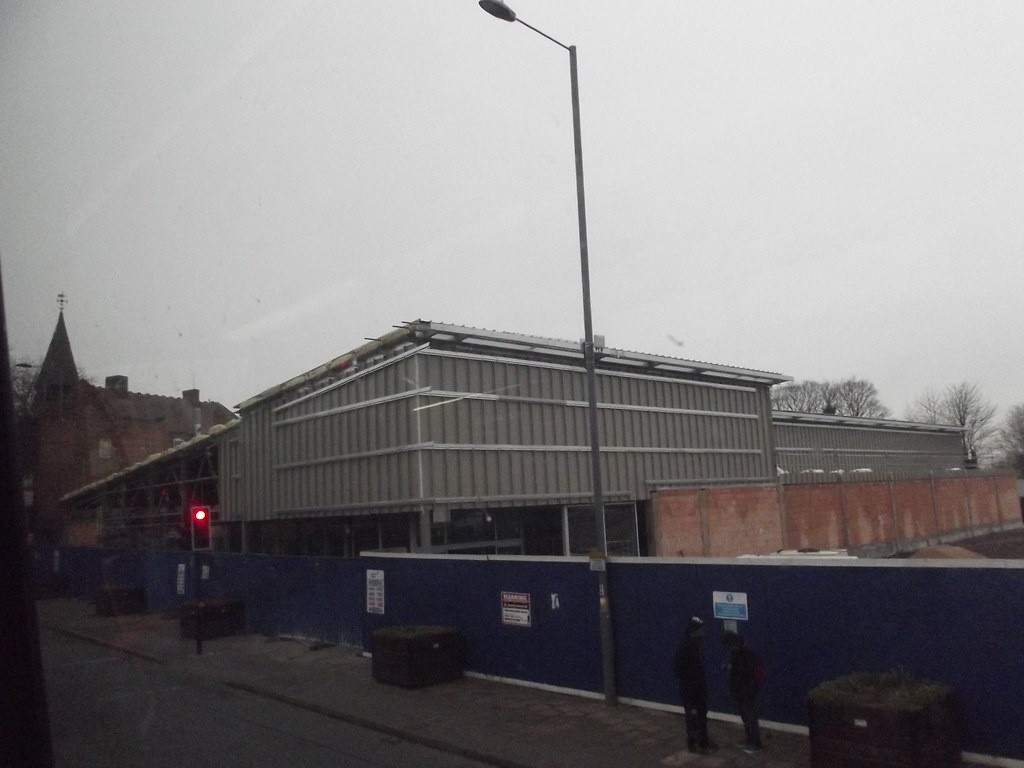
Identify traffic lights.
[190,505,211,551]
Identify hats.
[686,616,704,633]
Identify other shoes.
[736,742,764,753]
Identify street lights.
[478,0,622,709]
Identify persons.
[718,630,765,753]
[673,617,719,754]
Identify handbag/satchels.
[753,662,766,687]
